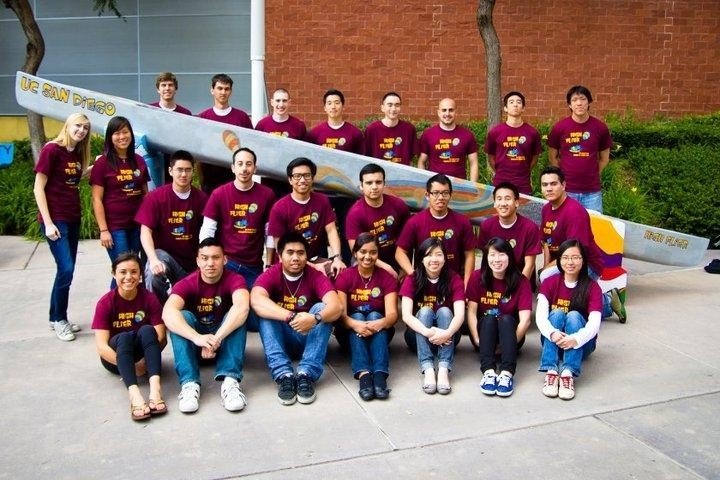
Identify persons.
[135,72,627,324]
[535,239,603,399]
[334,232,399,401]
[249,232,344,405]
[91,253,168,421]
[465,237,532,397]
[398,236,466,395]
[161,237,251,413]
[89,116,151,290]
[33,112,102,342]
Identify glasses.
[430,191,449,199]
[292,172,313,182]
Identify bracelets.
[100,230,108,232]
[549,333,556,341]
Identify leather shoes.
[422,369,436,396]
[436,370,452,396]
[360,375,389,401]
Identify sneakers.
[276,377,296,407]
[177,383,200,413]
[220,380,247,412]
[497,370,514,397]
[559,369,575,401]
[48,320,81,342]
[296,374,316,405]
[542,369,559,398]
[479,370,495,397]
[608,289,626,324]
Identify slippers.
[149,391,167,417]
[132,401,151,422]
